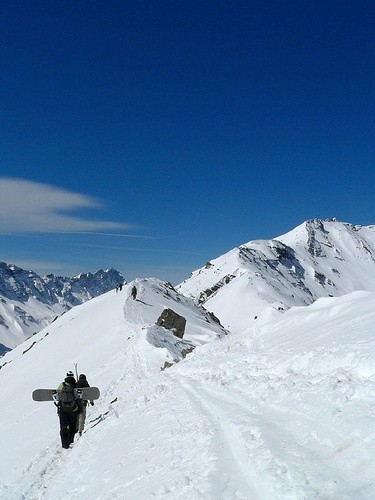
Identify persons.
[56,370,83,449]
[75,374,94,437]
[131,286,137,300]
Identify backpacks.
[61,382,78,413]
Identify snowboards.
[32,387,100,401]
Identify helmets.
[79,374,86,380]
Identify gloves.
[90,402,94,406]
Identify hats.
[66,371,74,377]
